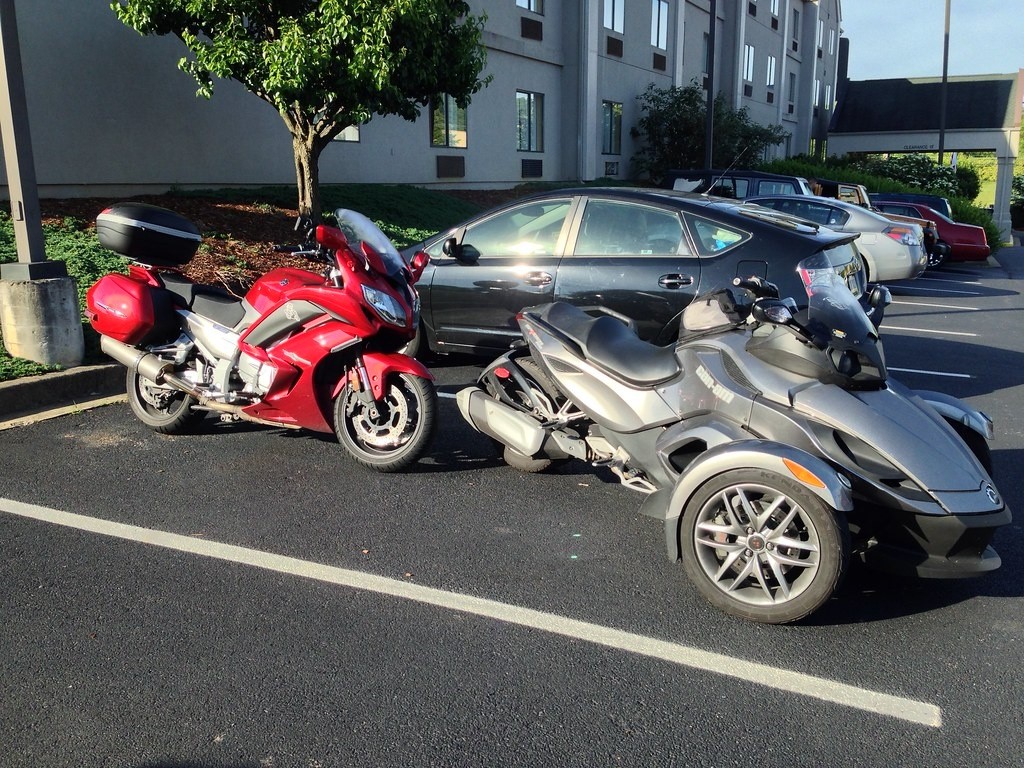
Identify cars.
[645,194,929,283]
[672,167,840,224]
[807,178,939,253]
[328,188,893,357]
[870,201,991,271]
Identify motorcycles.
[86,202,440,473]
[456,273,1014,625]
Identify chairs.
[575,207,614,256]
